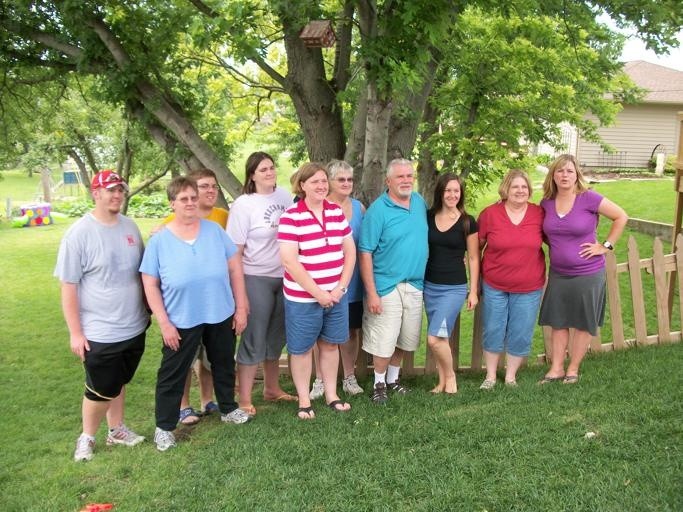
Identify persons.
[358,157,429,403]
[475,168,545,388]
[539,155,628,386]
[225,153,298,418]
[309,159,367,400]
[55,172,151,462]
[138,176,248,453]
[425,173,480,394]
[151,170,229,426]
[277,163,358,419]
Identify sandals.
[370,383,389,405]
[385,381,414,403]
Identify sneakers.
[310,380,325,401]
[75,433,97,463]
[105,426,145,449]
[341,376,364,397]
[154,427,178,453]
[220,410,251,426]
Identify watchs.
[336,282,348,295]
[603,240,613,251]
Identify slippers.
[196,402,221,419]
[240,404,257,418]
[564,374,580,390]
[263,394,301,405]
[178,406,201,427]
[536,374,565,387]
[295,407,318,422]
[327,400,353,413]
[480,379,496,391]
[504,380,519,390]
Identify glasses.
[331,176,354,182]
[175,197,199,203]
[197,183,221,192]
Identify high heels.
[445,373,457,393]
[431,383,445,393]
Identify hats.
[89,172,132,193]
